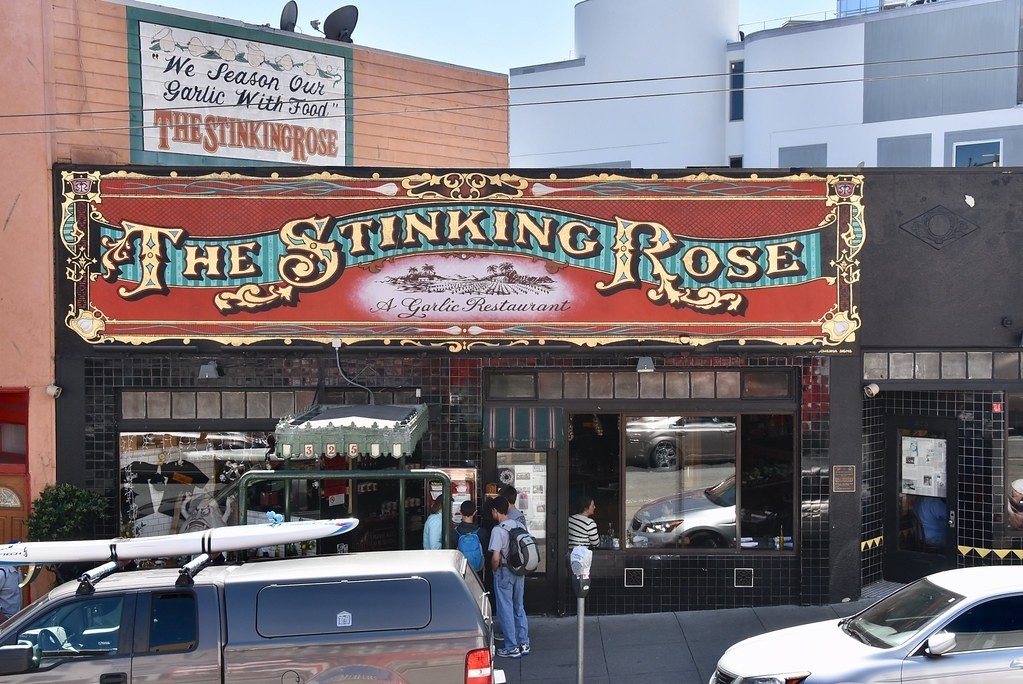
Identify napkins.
[734,537,758,547]
[773,537,793,546]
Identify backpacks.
[454,526,486,573]
[498,521,540,577]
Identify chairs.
[908,509,950,554]
[151,595,196,650]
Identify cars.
[624,414,737,472]
[628,468,875,549]
[707,564,1023,684]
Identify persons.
[648,503,679,548]
[912,495,947,550]
[424,486,530,657]
[0,540,24,625]
[568,496,599,550]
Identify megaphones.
[46,385,62,399]
[864,383,879,397]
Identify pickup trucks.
[0,517,507,684]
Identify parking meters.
[569,545,594,684]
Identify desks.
[743,536,776,548]
[597,536,618,549]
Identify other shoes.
[494,632,505,641]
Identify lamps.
[636,357,655,373]
[198,361,220,379]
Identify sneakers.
[520,643,531,655]
[497,647,521,658]
